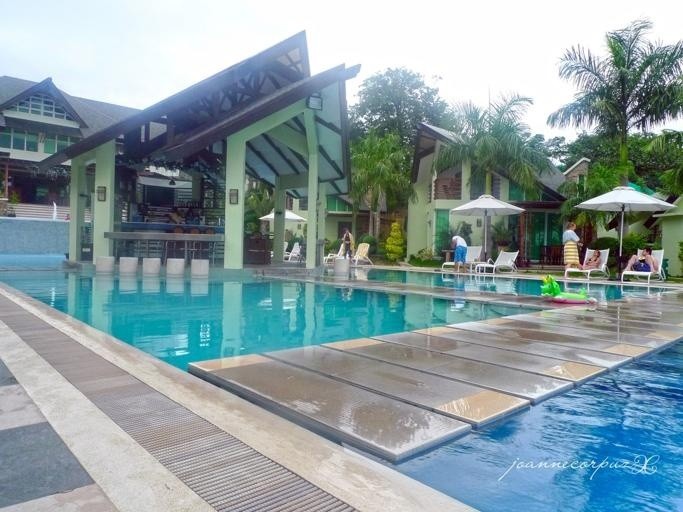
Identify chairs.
[324,242,374,267]
[442,246,520,274]
[620,248,667,284]
[565,247,610,281]
[168,226,215,259]
[271,241,301,262]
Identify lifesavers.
[541,276,598,305]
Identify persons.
[625,246,658,272]
[565,220,581,277]
[451,236,467,272]
[186,210,199,223]
[577,251,599,270]
[168,207,182,223]
[342,227,355,258]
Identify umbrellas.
[259,209,306,241]
[573,186,680,271]
[451,195,525,262]
